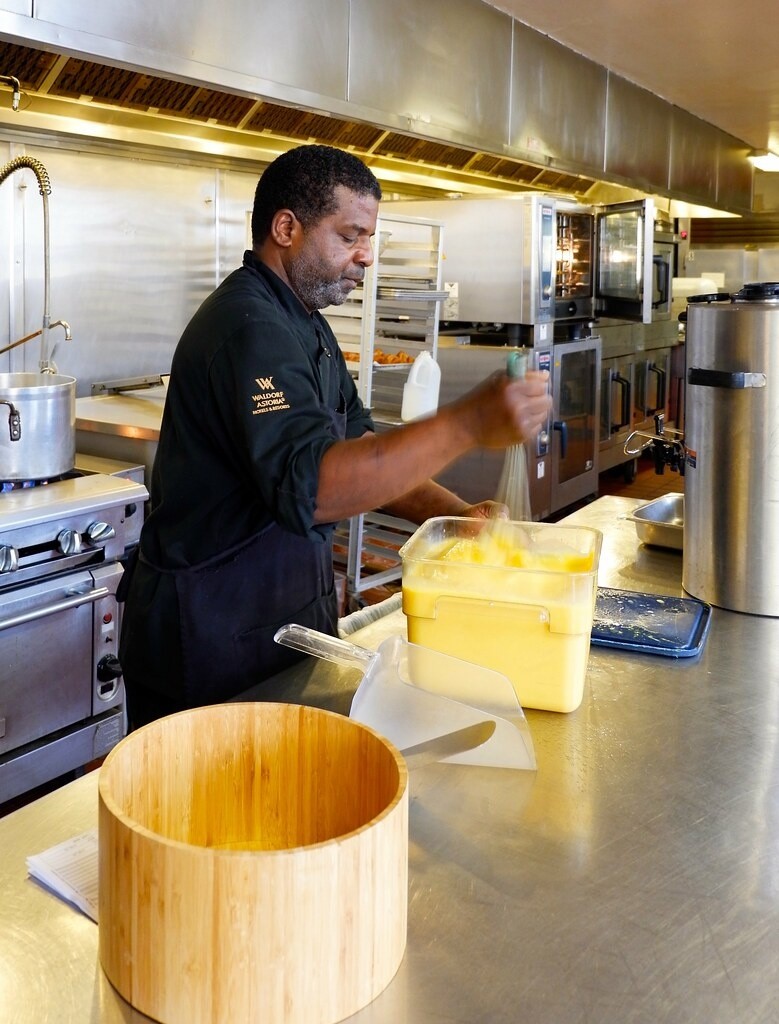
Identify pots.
[0,372,77,483]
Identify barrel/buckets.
[622,283,778,622]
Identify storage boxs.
[399,514,604,716]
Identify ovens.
[378,197,654,521]
[0,563,129,805]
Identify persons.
[114,145,553,735]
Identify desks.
[0,496,779,1024]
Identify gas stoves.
[0,468,150,577]
